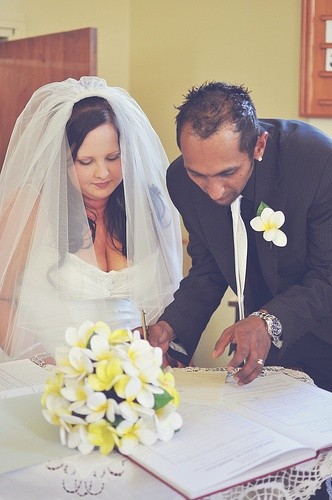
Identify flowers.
[41,320,183,457]
[250,200,288,249]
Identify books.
[114,374,332,499]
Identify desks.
[0,367,332,500]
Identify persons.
[0,77,195,367]
[132,82,331,392]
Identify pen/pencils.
[141,310,148,341]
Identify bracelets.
[31,352,53,366]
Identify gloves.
[40,364,68,379]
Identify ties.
[231,195,247,366]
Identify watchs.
[249,312,283,349]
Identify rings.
[257,358,266,368]
